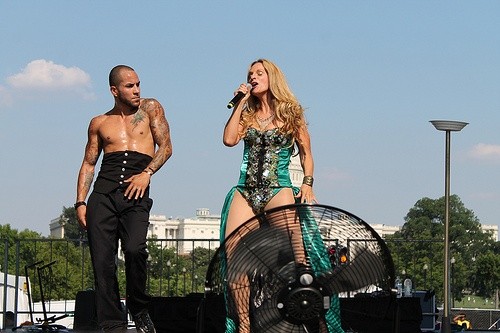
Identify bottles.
[395,276,402,298]
[404,276,413,297]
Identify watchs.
[74,201,87,211]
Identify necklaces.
[254,112,277,131]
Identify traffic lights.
[339,247,348,264]
[328,247,337,269]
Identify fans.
[203,201,397,333]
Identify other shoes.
[134,312,156,333]
[99,323,129,333]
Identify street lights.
[401,269,406,297]
[422,264,428,291]
[146,253,152,295]
[182,266,186,295]
[429,120,469,333]
[450,257,456,308]
[167,260,171,290]
[194,275,198,294]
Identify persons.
[74,65,172,333]
[452,313,472,331]
[219,59,329,333]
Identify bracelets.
[303,175,313,187]
[143,166,155,175]
